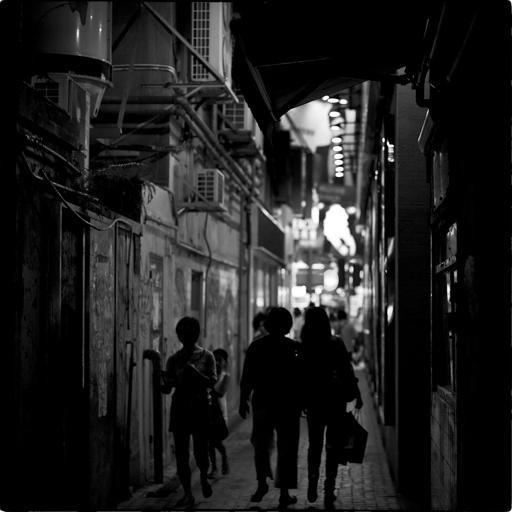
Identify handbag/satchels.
[340,411,368,467]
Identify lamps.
[322,95,359,179]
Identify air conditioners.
[193,168,226,212]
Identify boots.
[307,478,337,509]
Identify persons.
[239,303,362,505]
[163,317,218,506]
[207,349,229,478]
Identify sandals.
[177,481,212,506]
[251,483,297,507]
[208,462,229,477]
